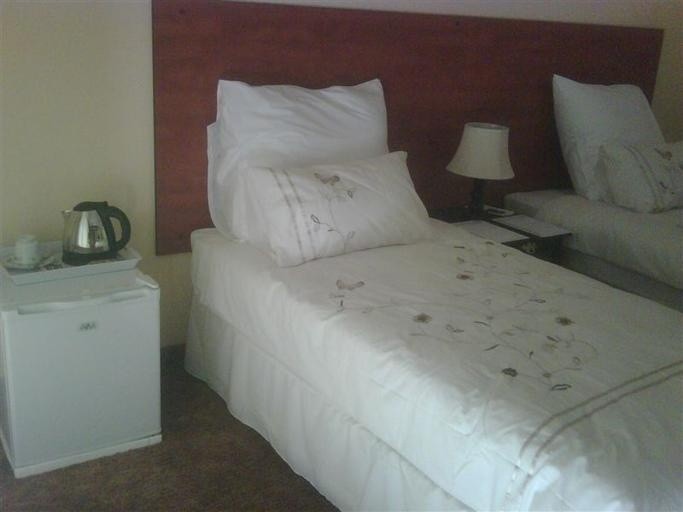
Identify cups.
[11,234,41,266]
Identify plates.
[3,253,53,270]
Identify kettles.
[58,200,132,264]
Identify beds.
[184,216,683,512]
[503,188,683,311]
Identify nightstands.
[428,204,572,264]
[0,268,162,480]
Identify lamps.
[445,120,515,220]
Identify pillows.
[248,150,442,267]
[601,141,683,213]
[205,77,389,242]
[551,74,665,201]
